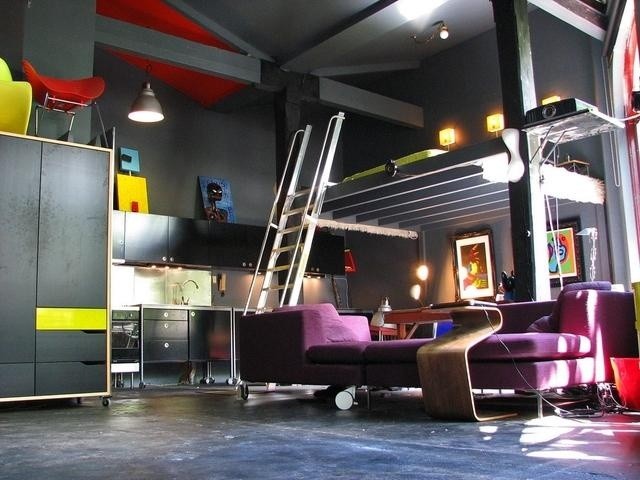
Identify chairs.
[20,58,109,149]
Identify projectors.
[524,99,598,125]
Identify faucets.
[177,279,200,305]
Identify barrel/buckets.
[609,357,640,409]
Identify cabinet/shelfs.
[110,209,345,276]
[109,308,376,389]
[0,130,115,410]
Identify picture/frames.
[449,217,585,305]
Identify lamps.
[127,60,166,123]
[408,20,450,46]
[438,95,561,147]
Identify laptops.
[431,299,498,309]
